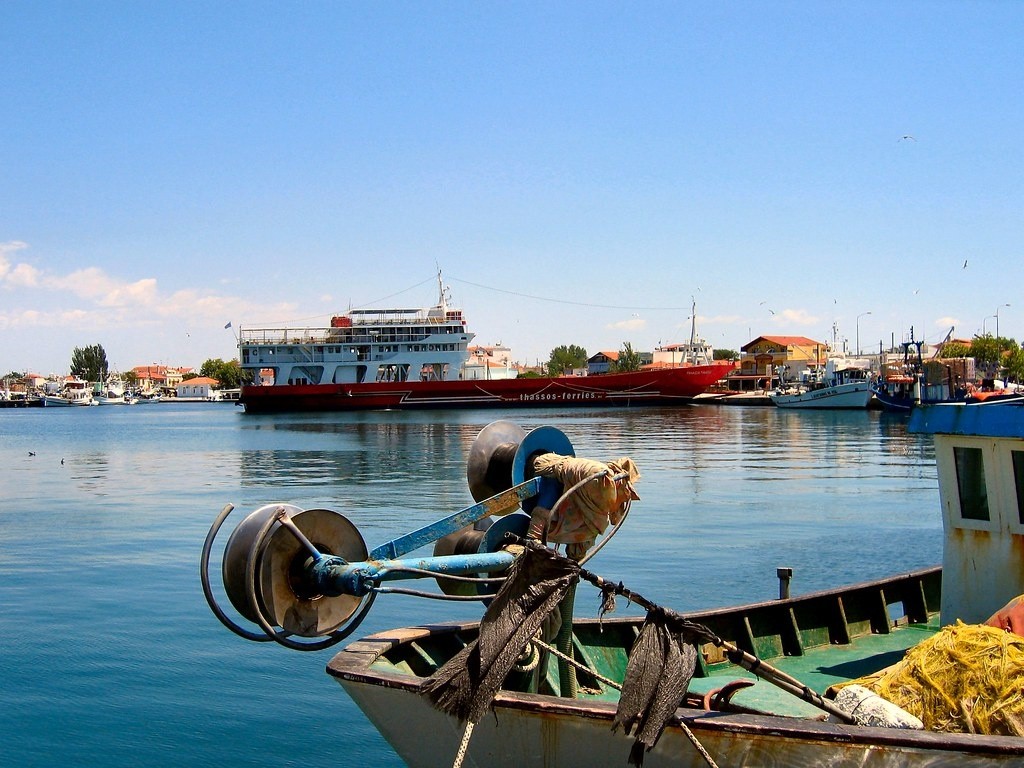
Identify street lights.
[856,312,872,359]
[997,303,1011,364]
[983,315,996,347]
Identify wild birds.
[962,260,968,268]
[28,451,36,455]
[897,135,918,142]
[61,457,66,464]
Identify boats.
[196,390,1024,768]
[0,376,162,408]
[769,320,870,410]
[872,325,1021,414]
[224,257,737,416]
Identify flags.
[225,322,232,329]
